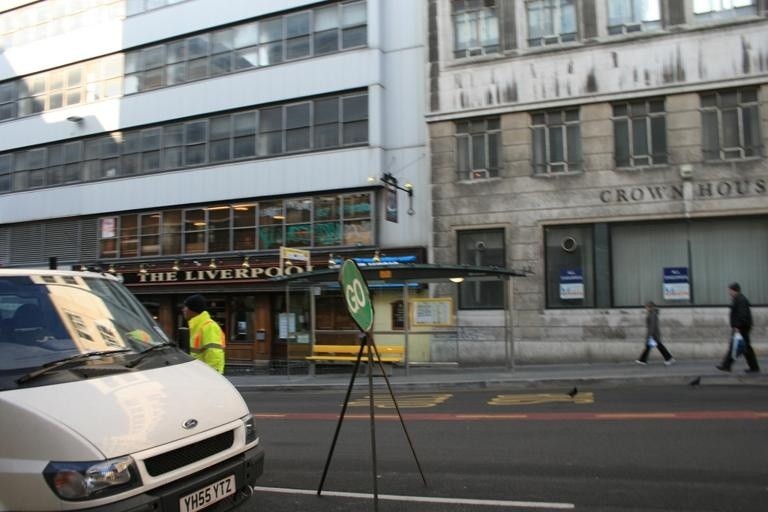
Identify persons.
[634,300,677,366]
[714,281,761,374]
[181,294,226,376]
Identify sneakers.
[717,365,730,372]
[664,358,675,366]
[745,367,759,372]
[636,359,648,366]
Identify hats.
[184,294,207,313]
[728,284,740,292]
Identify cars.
[0,268,264,512]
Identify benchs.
[305,345,404,379]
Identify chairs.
[13,305,45,341]
